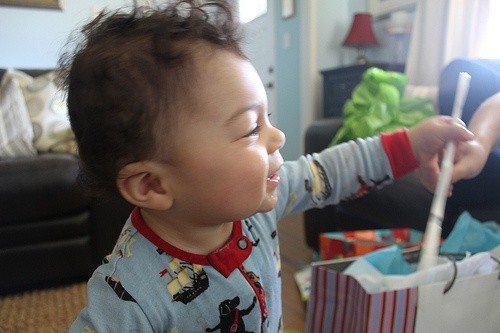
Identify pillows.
[0,68,79,160]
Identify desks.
[320,61,406,118]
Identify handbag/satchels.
[306,250,500,333]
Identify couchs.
[0,67,129,296]
[303,59,500,254]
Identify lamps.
[343,13,379,66]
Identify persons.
[414,93,500,198]
[66,1,474,333]
